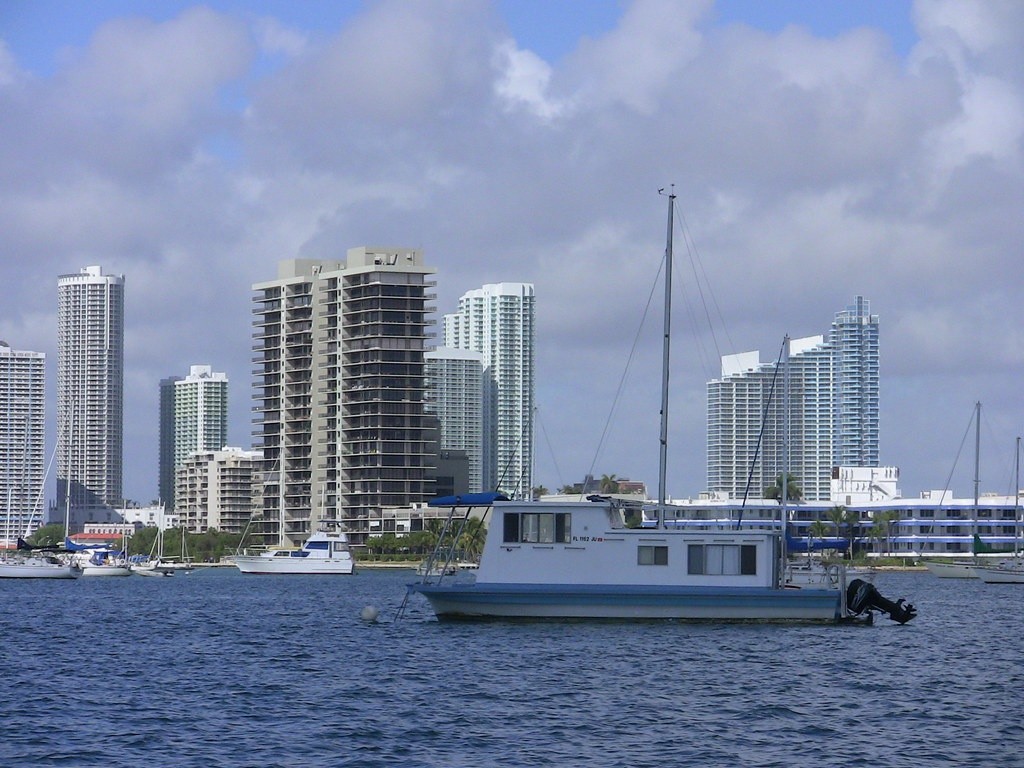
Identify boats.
[221,483,356,576]
[0,401,197,578]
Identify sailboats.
[914,399,1001,579]
[971,435,1024,584]
[406,186,919,629]
